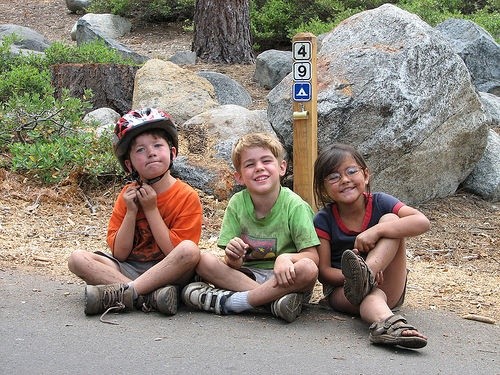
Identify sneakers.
[270,293,303,324]
[84,282,137,316]
[181,282,235,315]
[138,285,179,317]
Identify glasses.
[323,167,363,184]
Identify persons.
[179,131,320,322]
[321,143,431,349]
[67,107,203,325]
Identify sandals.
[341,249,377,305]
[369,315,429,348]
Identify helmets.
[112,107,178,158]
